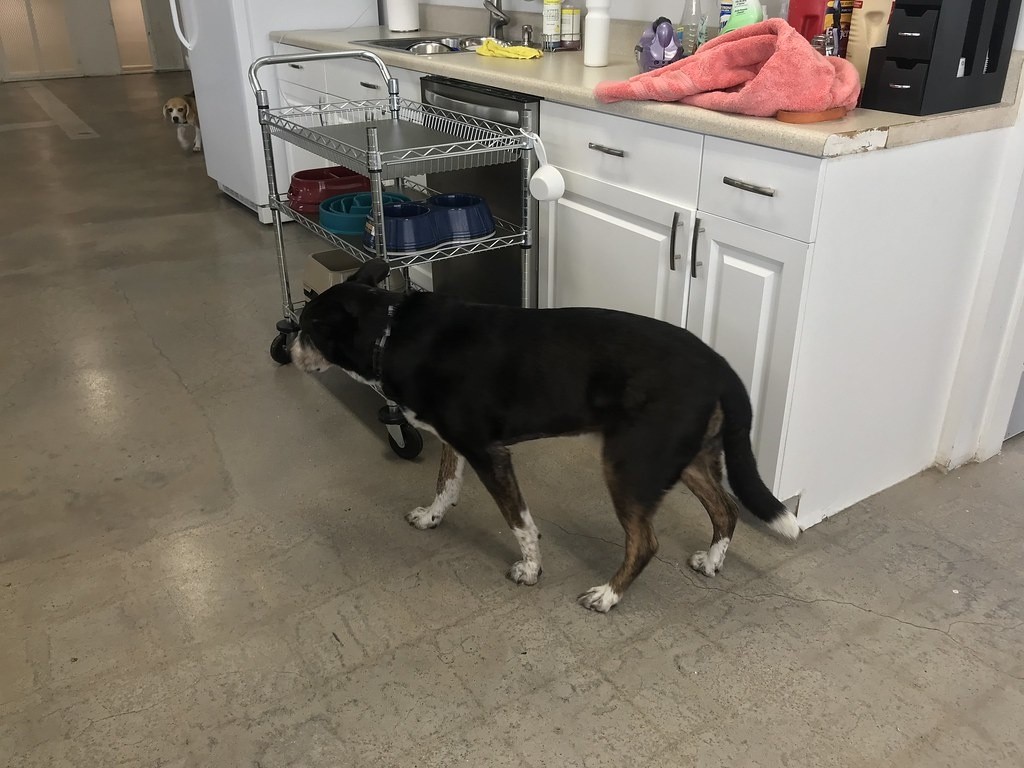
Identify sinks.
[348,32,541,57]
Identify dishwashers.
[420,74,540,306]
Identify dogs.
[291,258,801,614]
[162,90,203,152]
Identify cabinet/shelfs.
[267,27,1021,537]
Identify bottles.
[583,0,611,67]
[680,0,702,56]
[837,0,855,59]
[824,1,835,34]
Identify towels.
[593,18,862,119]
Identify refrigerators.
[168,0,379,226]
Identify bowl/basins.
[405,35,507,53]
